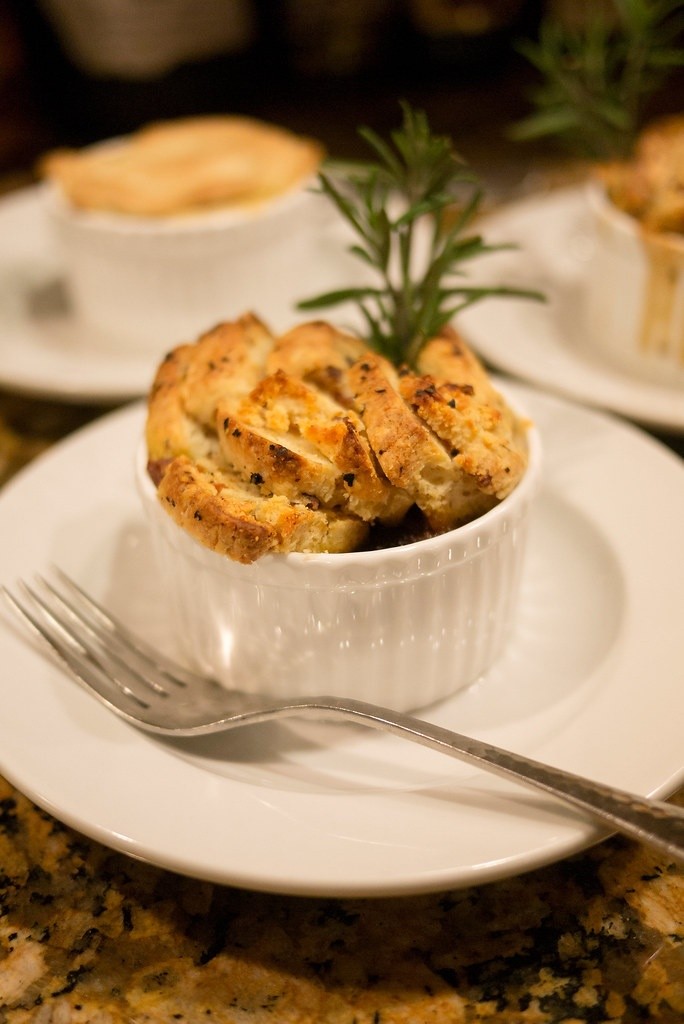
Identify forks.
[0,557,684,861]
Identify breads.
[605,111,684,244]
[36,114,324,219]
[142,310,523,562]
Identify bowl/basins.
[49,182,308,333]
[582,170,684,388]
[131,382,544,724]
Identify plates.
[0,374,684,897]
[437,182,684,434]
[1,163,434,405]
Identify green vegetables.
[497,0,684,166]
[293,104,549,367]
